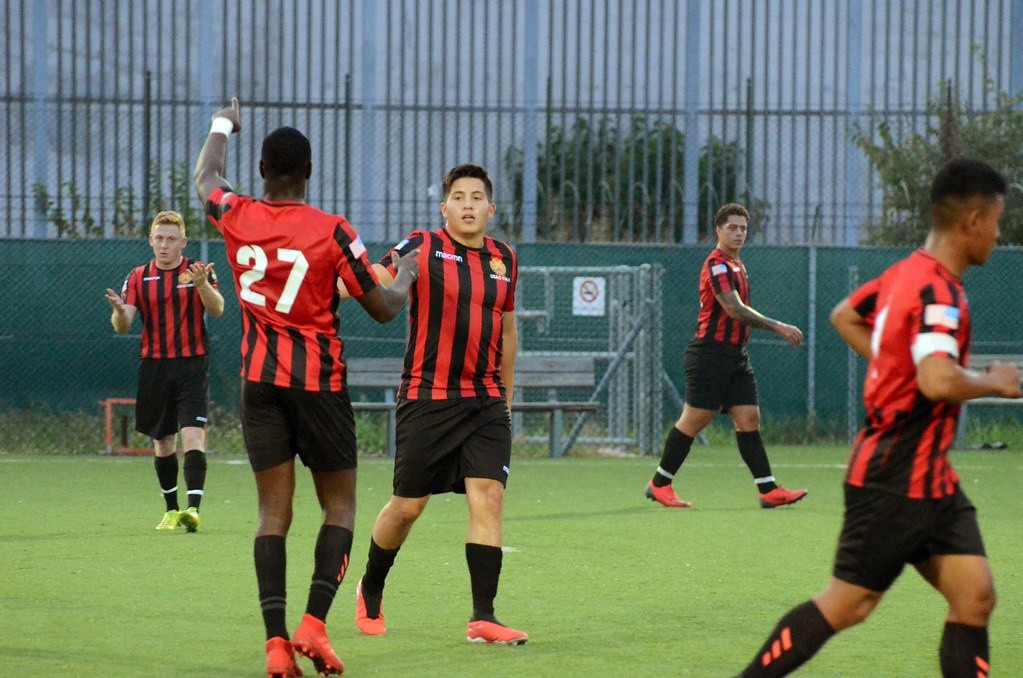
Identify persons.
[340,164,528,647]
[193,98,421,678]
[104,212,225,533]
[645,203,808,509]
[744,158,1023,678]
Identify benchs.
[955,353,1023,451]
[345,353,600,457]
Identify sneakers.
[355,578,386,635]
[179,507,200,531]
[291,614,345,677]
[156,509,183,530]
[265,637,305,678]
[645,480,692,507]
[759,485,808,508]
[466,618,528,646]
